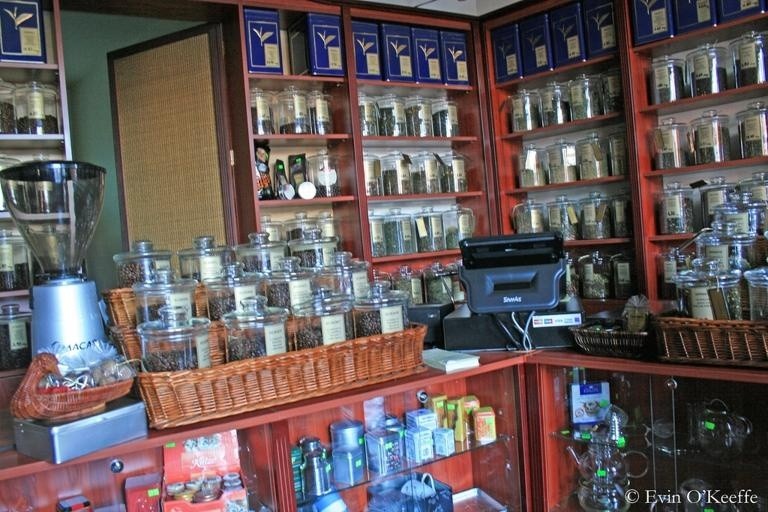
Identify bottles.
[110,226,413,384]
[302,439,331,494]
[353,90,479,304]
[1,73,67,378]
[247,79,342,134]
[503,65,639,300]
[298,144,346,197]
[645,34,768,330]
[257,208,347,246]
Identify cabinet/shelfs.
[219,1,500,283]
[475,1,768,321]
[0,344,532,512]
[524,348,768,511]
[0,0,75,449]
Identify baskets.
[656,307,766,368]
[12,348,136,422]
[571,311,658,359]
[100,270,350,327]
[137,321,427,430]
[112,305,374,367]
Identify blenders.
[0,152,118,387]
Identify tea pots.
[567,390,764,512]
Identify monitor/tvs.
[458,230,565,312]
